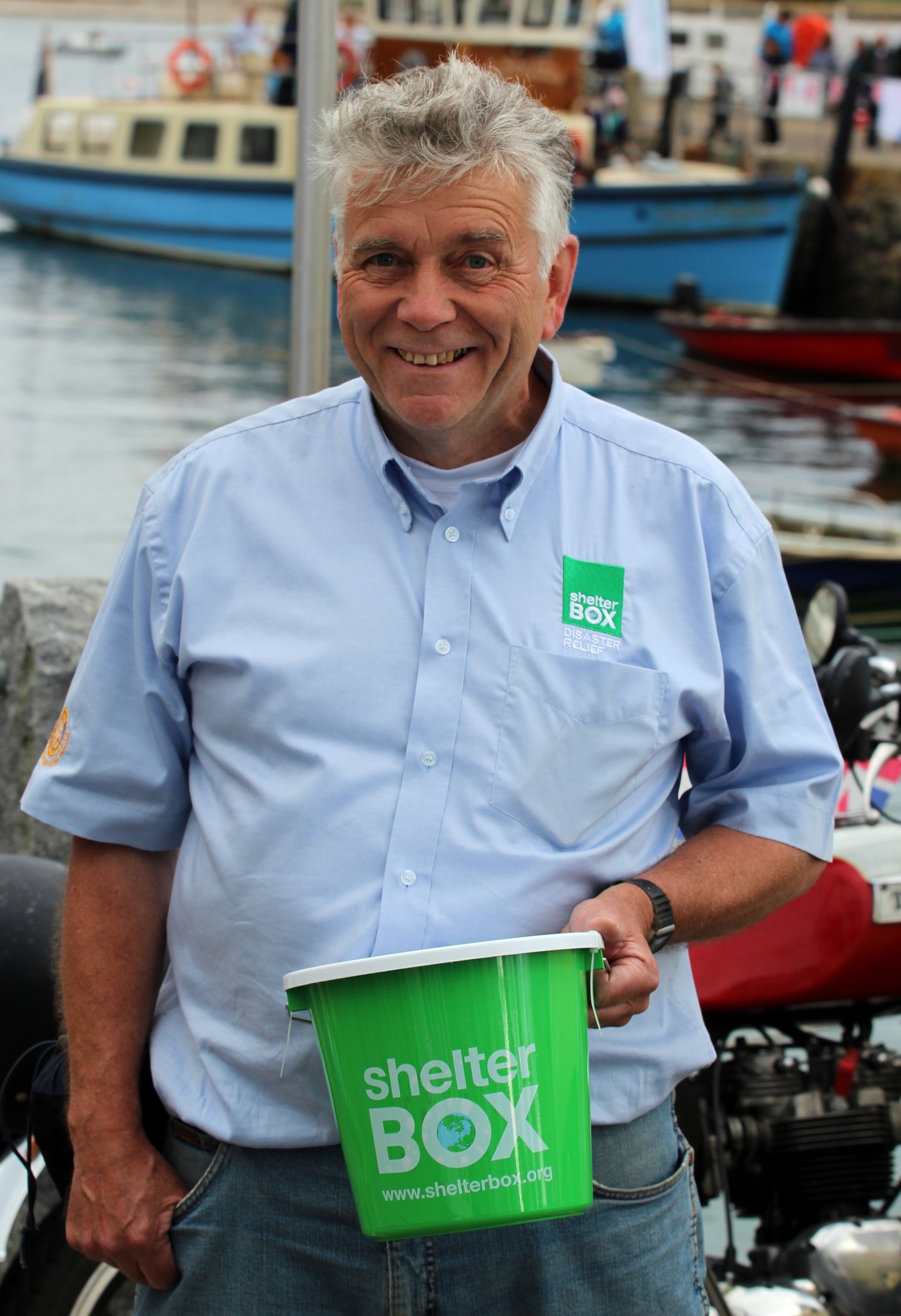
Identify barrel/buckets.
[282,930,606,1243]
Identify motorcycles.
[0,580,901,1316]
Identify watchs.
[626,878,675,953]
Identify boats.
[840,403,901,467]
[0,0,814,318]
[655,274,900,385]
[751,496,901,643]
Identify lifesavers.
[337,42,358,91]
[166,39,215,93]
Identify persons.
[763,68,780,143]
[812,38,838,80]
[19,62,843,1316]
[708,64,732,140]
[224,5,266,55]
[590,85,627,155]
[590,5,627,72]
[337,7,372,59]
[857,36,891,76]
[764,11,793,67]
